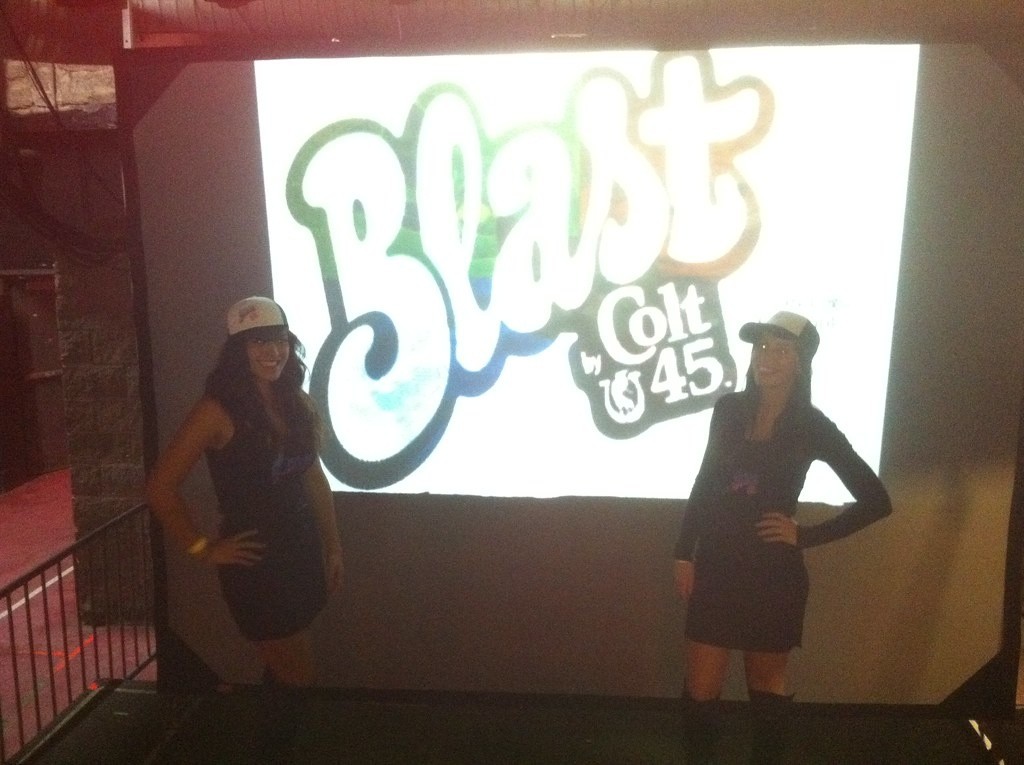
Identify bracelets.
[185,537,207,554]
[675,559,692,564]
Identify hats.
[736,310,819,360]
[226,295,289,335]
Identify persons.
[145,296,346,765]
[666,311,893,765]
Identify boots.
[251,670,308,765]
[683,677,719,765]
[746,688,796,765]
